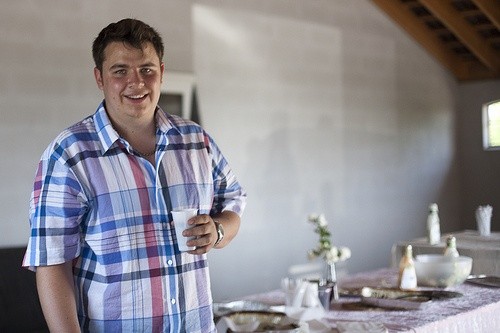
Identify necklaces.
[137,150,155,155]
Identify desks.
[391,230,500,276]
[214,265,500,333]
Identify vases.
[326,262,338,301]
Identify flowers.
[308,212,351,263]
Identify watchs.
[214,221,224,248]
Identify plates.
[465,274,500,287]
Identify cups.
[170,208,198,252]
[302,283,319,307]
[280,288,305,307]
[475,214,492,236]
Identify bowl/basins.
[414,254,473,288]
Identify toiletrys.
[396,244,417,291]
[425,202,441,246]
[443,236,459,257]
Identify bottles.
[397,244,417,291]
[425,204,441,243]
[444,235,459,257]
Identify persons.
[22,18,248,333]
